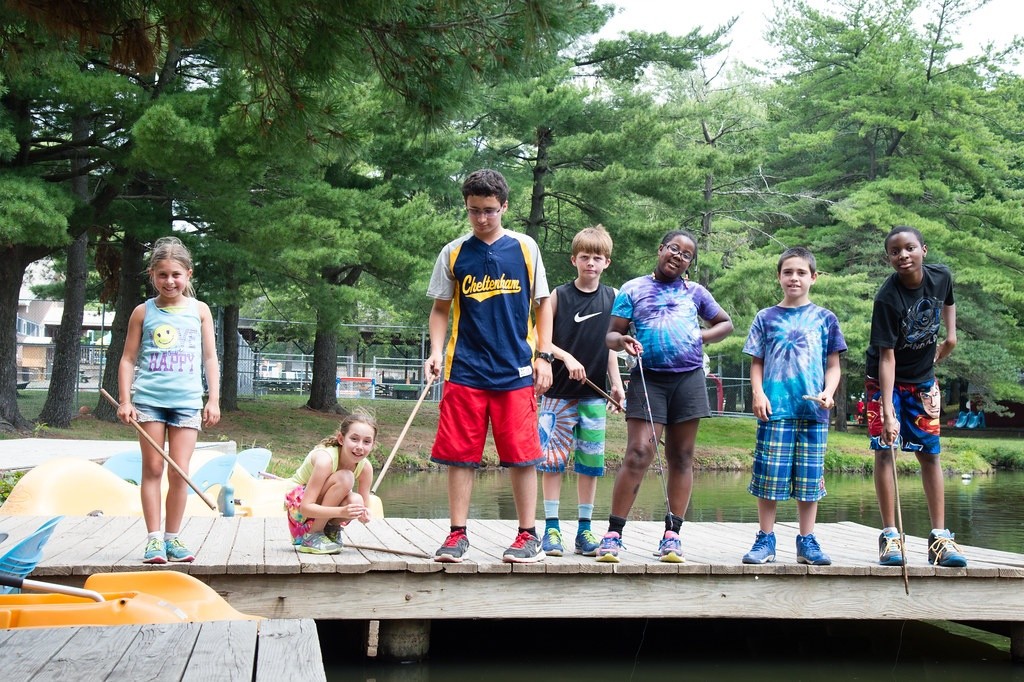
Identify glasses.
[662,242,693,264]
[464,200,504,218]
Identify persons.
[281,405,378,554]
[116,236,221,564]
[425,168,555,564]
[595,229,733,563]
[867,226,970,569]
[741,247,847,564]
[531,225,626,558]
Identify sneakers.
[878,529,908,565]
[542,527,571,556]
[433,527,471,562]
[502,531,548,562]
[795,532,831,566]
[595,530,628,563]
[325,524,344,549]
[575,530,600,554]
[928,527,968,566]
[166,537,195,562]
[658,531,684,562]
[743,529,777,563]
[144,539,168,565]
[299,531,341,554]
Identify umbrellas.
[95,331,113,346]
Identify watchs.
[534,350,555,363]
